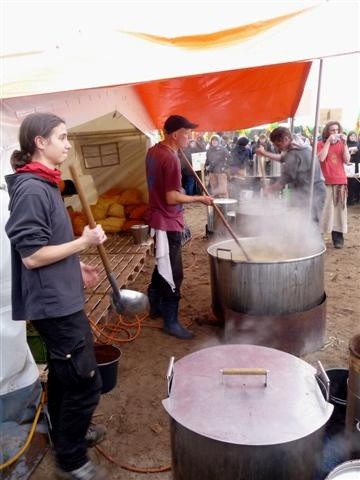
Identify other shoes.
[162,321,195,338]
[57,461,112,480]
[84,424,106,448]
[332,233,344,248]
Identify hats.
[164,115,199,134]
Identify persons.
[4,112,114,480]
[255,127,327,224]
[179,131,360,210]
[317,121,350,249]
[145,115,214,339]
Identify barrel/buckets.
[131,224,149,245]
[92,344,122,395]
[315,368,349,441]
[345,333,360,461]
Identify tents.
[132,56,323,218]
[57,109,161,213]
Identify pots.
[207,237,327,316]
[161,344,334,480]
[235,209,282,238]
[245,176,280,192]
